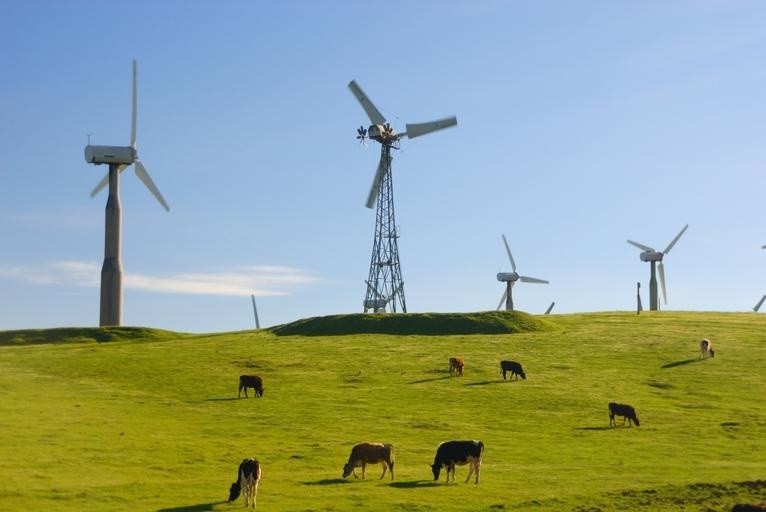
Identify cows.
[699,339,715,360]
[238,375,264,399]
[608,402,640,428]
[448,356,465,377]
[228,458,261,510]
[500,360,526,380]
[341,441,396,483]
[428,439,485,487]
